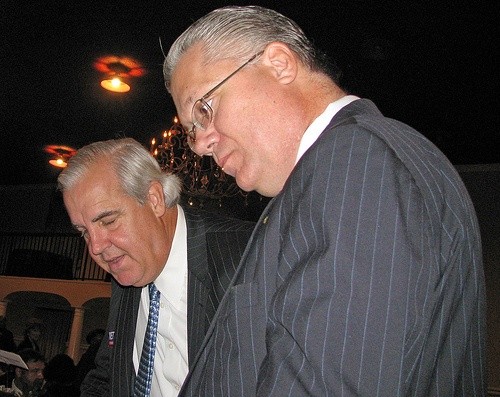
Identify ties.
[134,284,161,397]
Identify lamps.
[149,115,250,210]
[99,62,133,93]
[48,149,71,168]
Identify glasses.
[186,51,265,158]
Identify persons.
[0,315,106,397]
[57,137,258,397]
[159,5,488,397]
